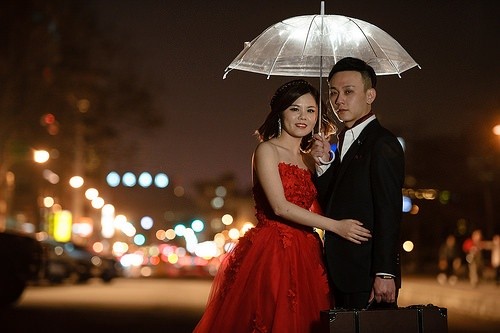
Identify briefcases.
[319,298,448,333]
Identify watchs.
[377,275,395,279]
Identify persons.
[311,55,406,309]
[191,79,373,333]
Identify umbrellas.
[224,0,422,164]
